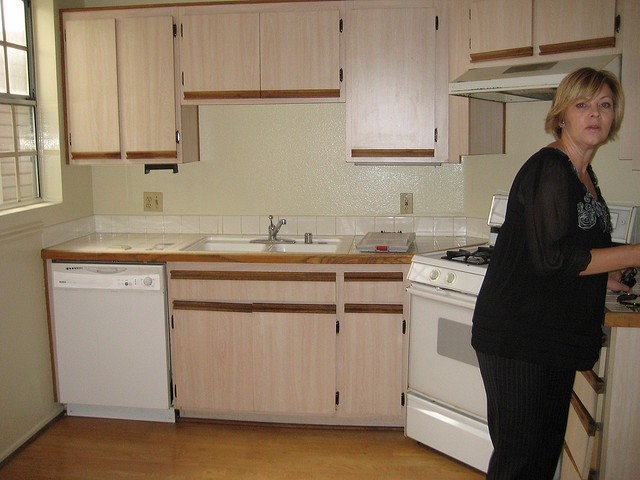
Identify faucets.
[250,215,295,243]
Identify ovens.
[404,284,502,475]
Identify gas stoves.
[408,241,500,296]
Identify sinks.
[179,235,341,254]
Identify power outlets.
[399,194,413,215]
[142,192,162,212]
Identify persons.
[471,68,639,480]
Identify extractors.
[445,54,620,102]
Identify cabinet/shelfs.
[58,3,199,166]
[468,2,622,65]
[559,290,640,480]
[167,254,339,433]
[343,255,408,432]
[338,2,509,166]
[175,5,347,106]
